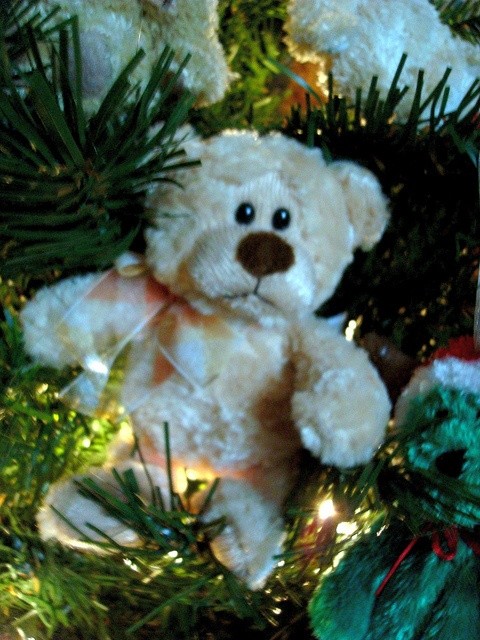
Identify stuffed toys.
[283,0,480,128]
[307,333,480,639]
[20,123,391,593]
[4,0,235,120]
